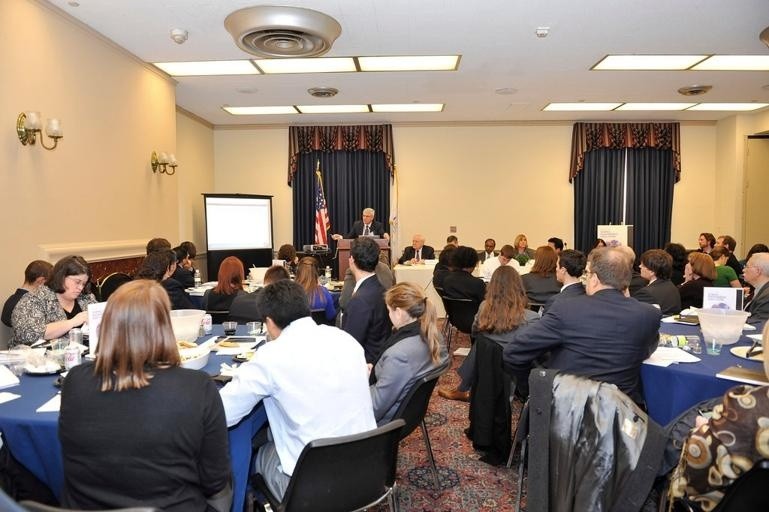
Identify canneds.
[202,314,213,335]
[68,328,82,347]
[64,347,81,369]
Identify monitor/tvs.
[343,232,358,239]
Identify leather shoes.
[437,386,471,402]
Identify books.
[35,390,63,414]
[0,364,21,391]
[0,346,48,365]
[713,365,769,387]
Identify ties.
[415,250,420,262]
[364,226,369,235]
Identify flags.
[314,174,331,245]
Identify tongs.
[215,335,257,343]
[746,341,764,358]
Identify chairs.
[245,347,452,512]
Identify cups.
[704,338,721,355]
[8,347,26,383]
[247,322,260,336]
[693,342,703,354]
[223,322,238,335]
[51,338,67,363]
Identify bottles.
[194,270,201,287]
[204,314,213,334]
[324,266,332,283]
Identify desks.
[640,309,768,428]
[393,259,537,318]
[0,323,269,511]
[184,279,344,325]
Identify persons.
[0,258,55,328]
[58,278,234,512]
[6,253,102,352]
[369,282,448,428]
[219,281,378,503]
[503,244,663,415]
[668,319,768,512]
[615,233,768,323]
[339,236,397,385]
[471,266,551,398]
[139,239,394,324]
[437,250,588,402]
[331,208,389,243]
[398,234,604,283]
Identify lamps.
[151,149,179,176]
[16,110,65,150]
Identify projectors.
[303,244,329,253]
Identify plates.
[24,364,60,374]
[190,281,218,297]
[745,334,764,343]
[730,347,765,362]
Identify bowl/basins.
[249,267,270,283]
[170,309,206,343]
[698,309,750,345]
[180,347,210,369]
